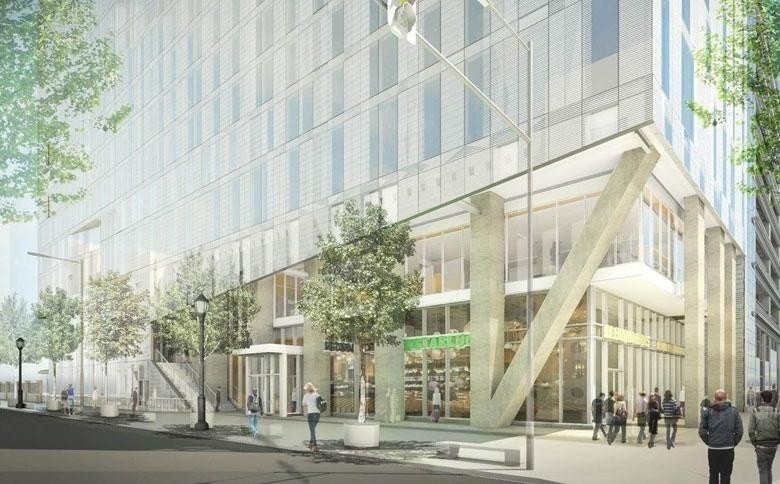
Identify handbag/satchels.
[638,414,646,425]
[612,416,626,426]
[675,407,680,417]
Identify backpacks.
[316,396,327,412]
[251,394,260,411]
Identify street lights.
[192,292,209,432]
[16,336,25,409]
[378,0,533,470]
[28,250,85,411]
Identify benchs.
[436,441,520,467]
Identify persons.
[432,386,442,421]
[589,387,683,451]
[303,381,328,453]
[131,387,138,411]
[91,386,99,410]
[247,387,265,438]
[698,382,780,484]
[59,381,75,416]
[214,386,222,412]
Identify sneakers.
[307,442,312,448]
[313,447,319,453]
[254,432,257,440]
